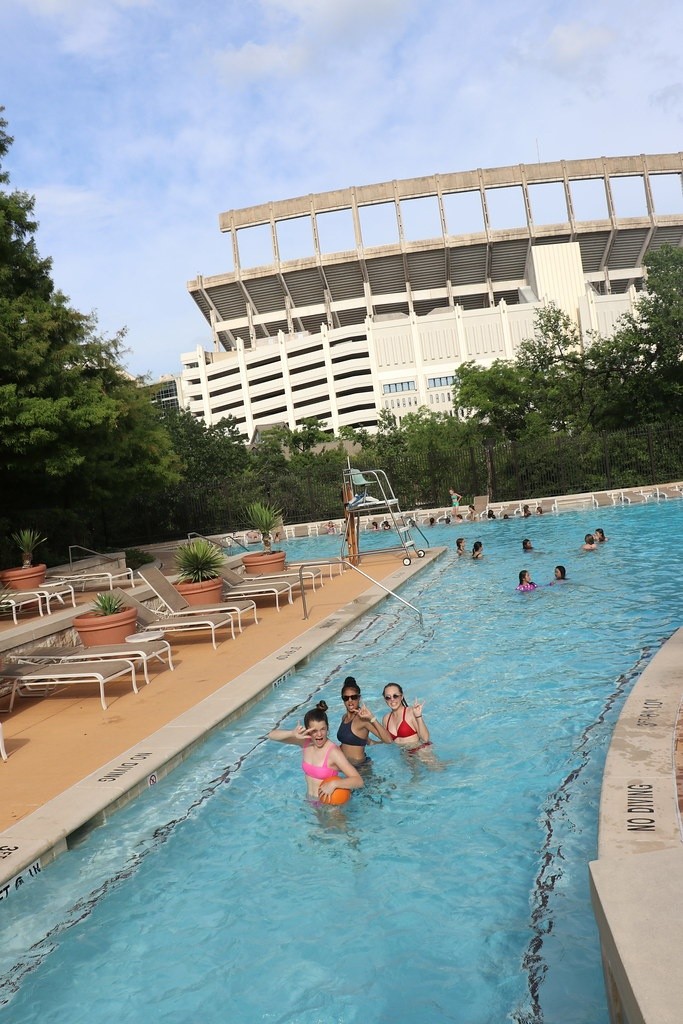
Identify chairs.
[232,484,683,545]
[0,558,343,761]
[351,469,377,486]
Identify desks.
[125,631,166,669]
[39,579,67,604]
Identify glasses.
[385,693,402,700]
[341,694,361,702]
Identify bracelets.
[416,716,422,718]
[370,717,377,724]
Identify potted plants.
[237,501,291,574]
[172,539,227,616]
[0,529,48,609]
[73,593,137,660]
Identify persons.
[487,510,494,521]
[274,532,281,542]
[455,514,463,523]
[554,566,568,581]
[516,570,538,591]
[536,507,543,515]
[523,505,532,516]
[582,534,597,550]
[372,521,379,530]
[449,489,463,515]
[504,514,508,519]
[445,518,451,526]
[322,521,337,535]
[469,505,479,522]
[592,528,608,543]
[268,700,366,805]
[428,518,435,527]
[522,539,535,551]
[457,538,466,553]
[471,541,483,558]
[352,682,429,746]
[383,521,391,530]
[334,677,393,766]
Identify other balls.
[318,776,351,805]
